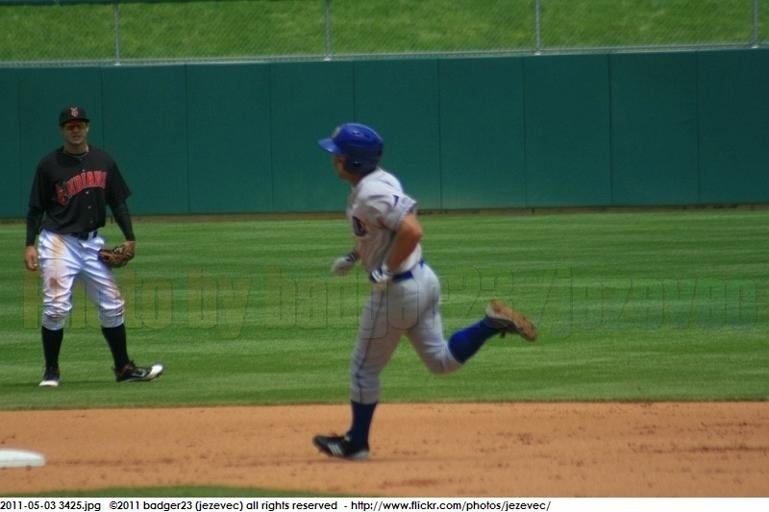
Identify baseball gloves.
[97,241,135,268]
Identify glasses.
[64,123,87,131]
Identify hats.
[58,105,90,124]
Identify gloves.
[329,251,361,277]
[368,261,394,286]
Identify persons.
[311,124,539,460]
[23,106,165,388]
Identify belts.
[45,225,98,241]
[393,259,424,284]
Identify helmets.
[316,121,385,176]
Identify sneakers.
[484,299,538,344]
[312,432,371,461]
[115,361,164,385]
[39,367,60,388]
